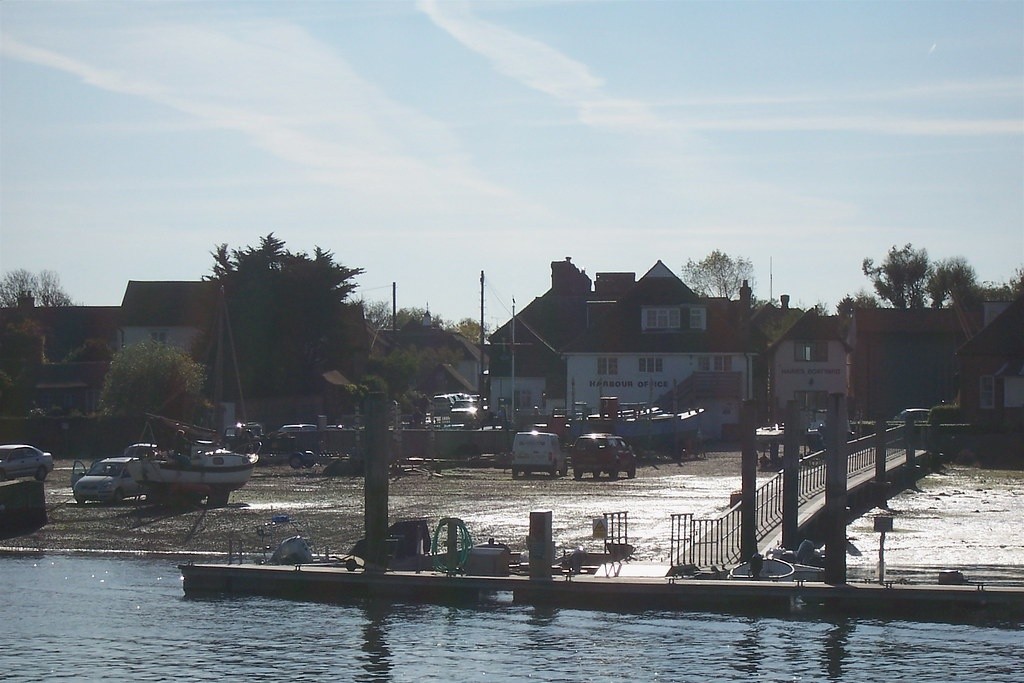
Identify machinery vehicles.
[144,411,317,469]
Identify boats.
[728,555,796,581]
[128,411,258,490]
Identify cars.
[892,408,931,424]
[0,445,55,483]
[71,458,144,506]
[433,392,481,425]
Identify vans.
[572,433,638,479]
[511,430,568,477]
